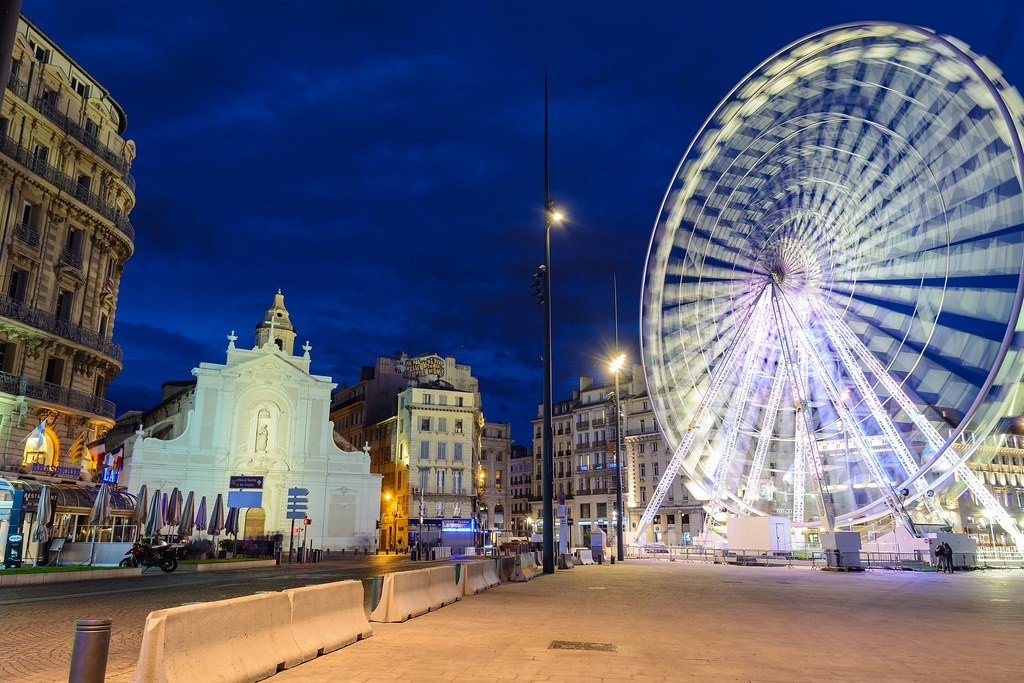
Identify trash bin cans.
[49,537,66,552]
[298,547,309,562]
[411,550,416,561]
[505,548,511,556]
[275,550,282,565]
[68,616,112,683]
[598,553,603,564]
[492,547,501,556]
[611,555,615,564]
[477,548,481,555]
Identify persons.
[936,542,953,573]
[155,530,163,543]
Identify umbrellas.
[225,507,239,555]
[89,483,111,542]
[132,484,147,540]
[196,497,207,540]
[178,491,195,538]
[32,486,51,563]
[168,487,183,544]
[145,489,164,544]
[162,492,168,526]
[207,494,225,554]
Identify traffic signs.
[229,476,264,489]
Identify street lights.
[609,353,626,561]
[527,518,531,541]
[530,200,578,574]
[984,509,998,560]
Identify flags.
[32,420,45,448]
[70,435,107,472]
[111,445,125,471]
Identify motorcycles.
[119,541,178,573]
